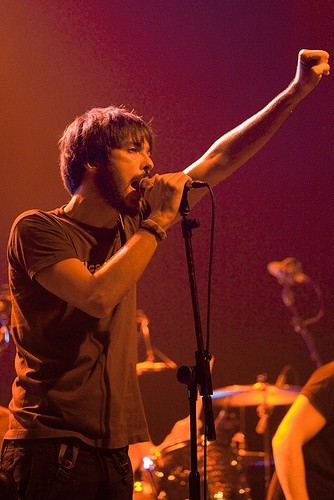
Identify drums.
[200,383,305,454]
[134,438,253,500]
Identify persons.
[0,49,329,500]
[266,362,334,500]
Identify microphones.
[255,366,291,435]
[138,177,208,197]
[266,261,312,285]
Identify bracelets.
[140,218,166,240]
